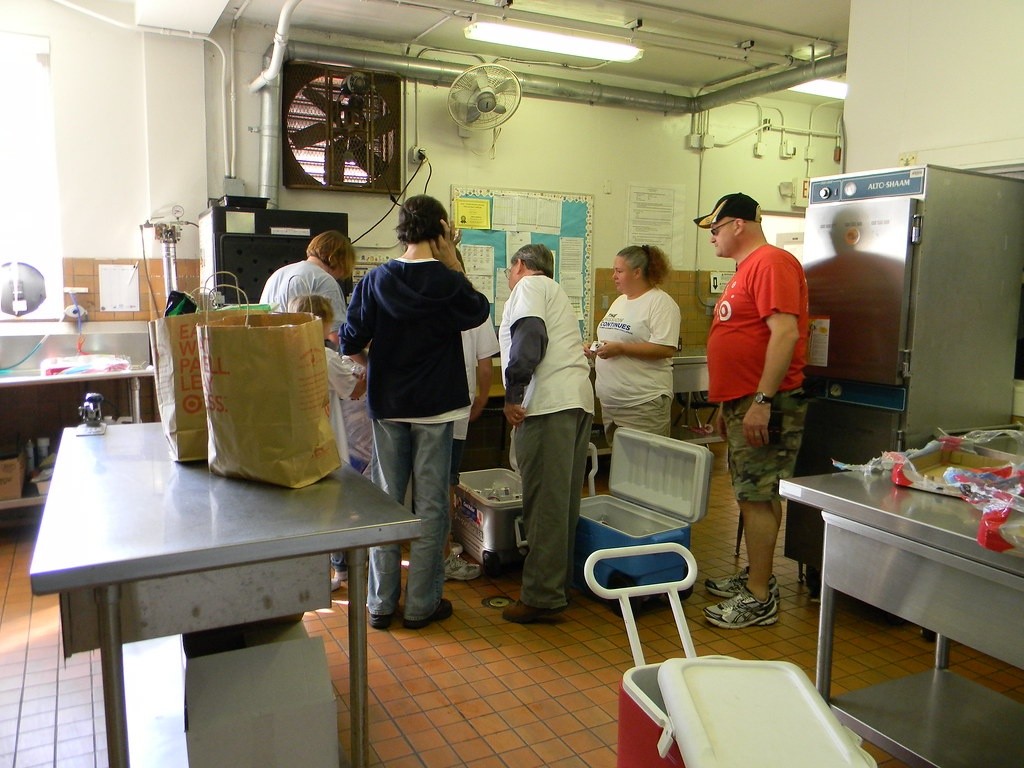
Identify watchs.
[752,391,774,404]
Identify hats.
[692,192,762,229]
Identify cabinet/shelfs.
[778,444,1024,768]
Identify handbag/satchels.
[148,271,268,463]
[195,275,341,489]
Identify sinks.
[672,356,710,393]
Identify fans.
[446,63,522,138]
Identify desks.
[0,366,154,509]
[30,421,421,768]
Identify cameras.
[589,341,605,352]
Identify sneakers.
[450,541,463,554]
[705,566,780,604]
[444,549,481,581]
[701,586,777,629]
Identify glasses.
[711,219,747,236]
[503,258,525,280]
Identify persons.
[288,294,368,592]
[694,193,809,628]
[338,194,490,630]
[498,243,595,622]
[584,245,682,450]
[258,230,368,367]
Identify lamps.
[787,77,849,101]
[465,22,645,63]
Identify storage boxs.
[32,468,51,495]
[895,432,1024,499]
[0,451,29,502]
[146,608,340,768]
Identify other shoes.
[335,567,348,580]
[369,611,393,628]
[564,586,572,601]
[402,598,453,630]
[502,599,567,625]
